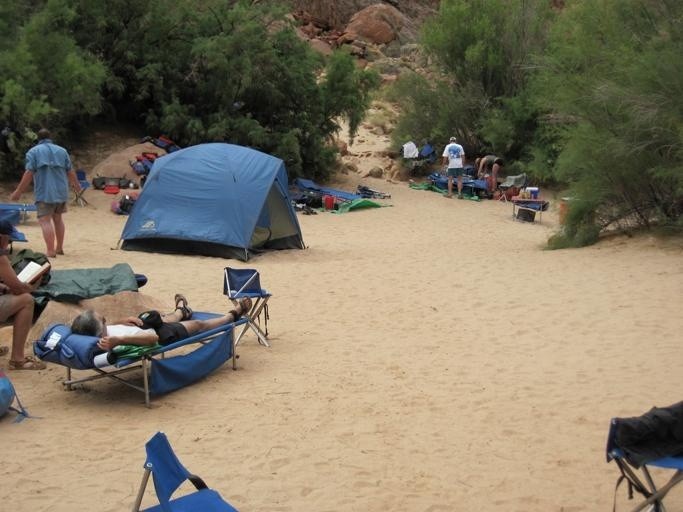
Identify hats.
[474,157,481,168]
[0,217,18,235]
[449,136,457,141]
[127,309,164,330]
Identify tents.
[116,142,308,257]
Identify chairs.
[219,267,274,342]
[66,166,91,206]
[424,170,490,200]
[126,433,239,511]
[0,201,35,221]
[296,174,362,208]
[36,301,254,406]
[607,425,682,512]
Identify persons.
[473,153,504,192]
[8,128,81,258]
[69,291,252,351]
[0,220,46,370]
[440,136,465,200]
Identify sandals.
[0,345,10,357]
[174,292,193,320]
[8,357,46,371]
[225,296,253,321]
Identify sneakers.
[443,193,453,198]
[457,194,464,199]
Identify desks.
[509,195,545,222]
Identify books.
[15,258,51,286]
